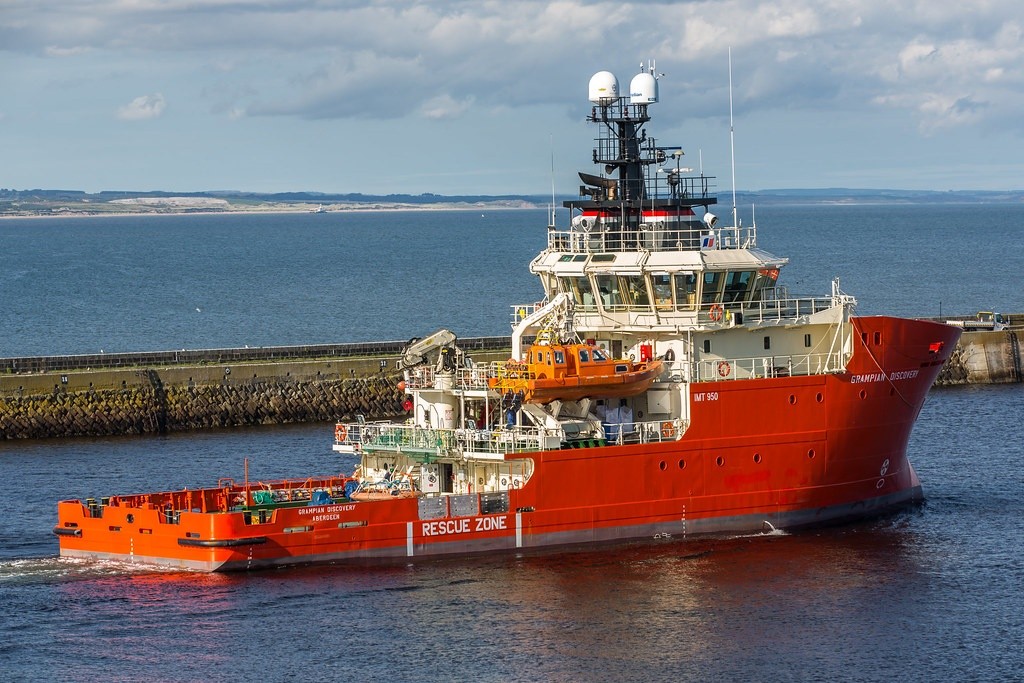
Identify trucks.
[946,312,1011,332]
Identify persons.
[222,482,233,512]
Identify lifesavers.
[719,361,730,376]
[662,423,675,436]
[335,426,346,441]
[710,304,721,322]
[664,348,676,365]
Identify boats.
[485,341,665,401]
[54,44,965,572]
